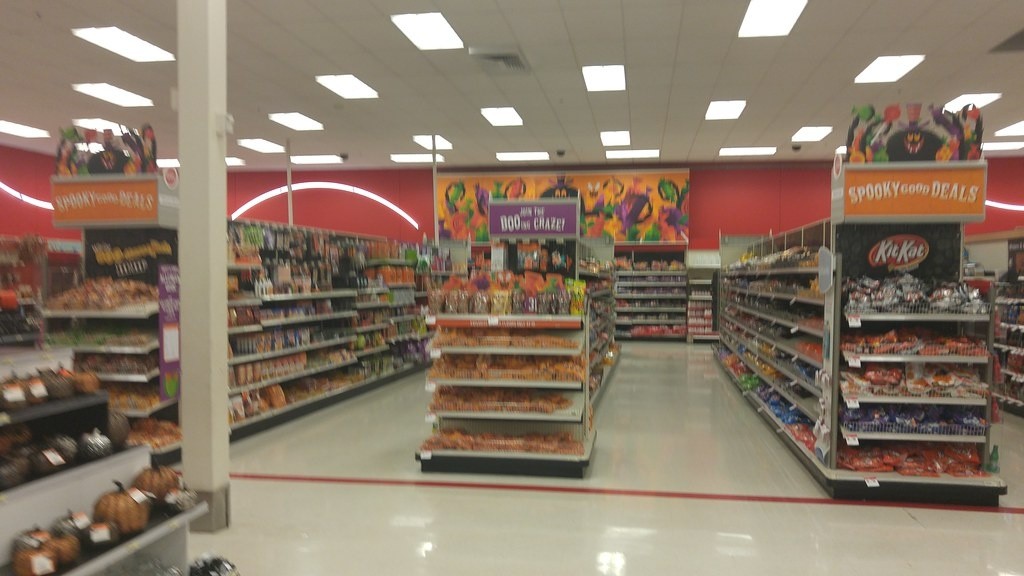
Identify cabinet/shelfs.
[38,218,432,444]
[414,240,622,479]
[993,235,1024,417]
[0,366,209,576]
[615,257,720,343]
[711,216,1009,507]
